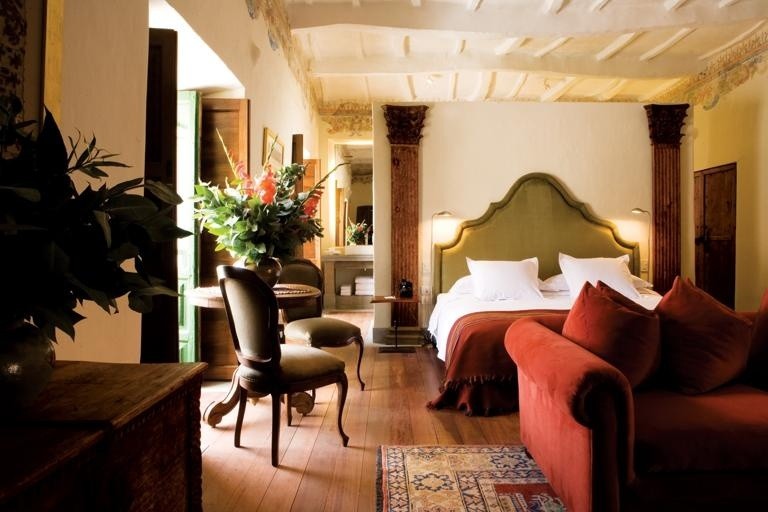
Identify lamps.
[630,206,653,284]
[423,208,452,350]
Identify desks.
[368,294,421,352]
[0,355,212,512]
[182,278,326,426]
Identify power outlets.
[419,285,432,305]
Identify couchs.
[501,303,767,512]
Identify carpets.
[374,438,568,512]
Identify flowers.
[342,216,372,247]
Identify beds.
[427,169,664,413]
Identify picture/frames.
[261,123,286,175]
[0,1,50,157]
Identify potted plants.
[188,122,351,262]
[0,88,200,403]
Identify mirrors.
[331,139,372,248]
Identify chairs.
[271,255,367,389]
[212,264,349,466]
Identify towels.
[338,276,373,297]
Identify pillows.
[447,274,544,299]
[464,252,545,302]
[567,281,767,399]
[557,250,641,301]
[541,271,653,292]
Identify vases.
[231,255,283,289]
